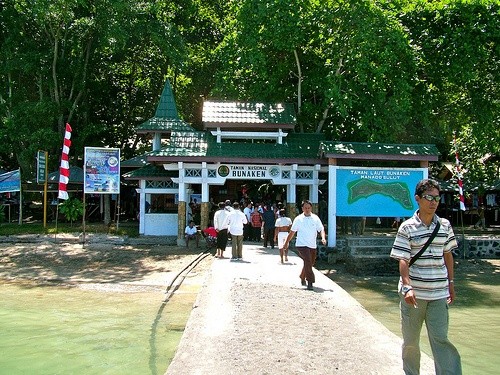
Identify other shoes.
[281,261,283,263]
[271,246,274,249]
[231,258,238,261]
[285,259,288,261]
[307,282,314,290]
[263,245,267,247]
[238,257,243,261]
[300,277,306,286]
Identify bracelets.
[450,280,454,282]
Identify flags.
[58,123,72,200]
[456,155,465,211]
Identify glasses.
[420,195,442,202]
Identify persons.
[240,201,282,249]
[274,210,292,264]
[204,198,218,249]
[188,197,201,224]
[224,200,233,245]
[283,200,327,290]
[226,203,248,261]
[214,202,230,257]
[390,179,462,375]
[137,201,150,219]
[185,221,199,250]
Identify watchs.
[402,286,413,295]
